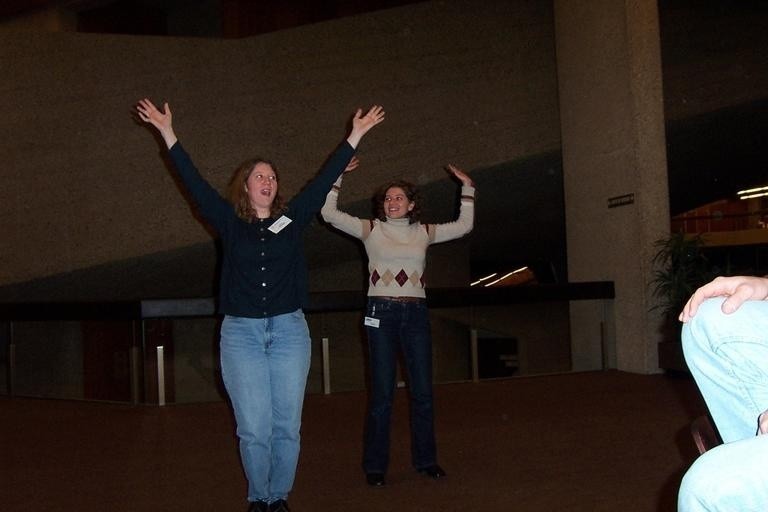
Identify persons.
[134,95,386,512]
[319,154,479,487]
[673,272,768,512]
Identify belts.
[369,296,426,304]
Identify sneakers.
[269,499,289,512]
[246,497,269,512]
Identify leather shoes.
[417,464,447,479]
[366,472,385,486]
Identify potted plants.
[646,227,724,369]
